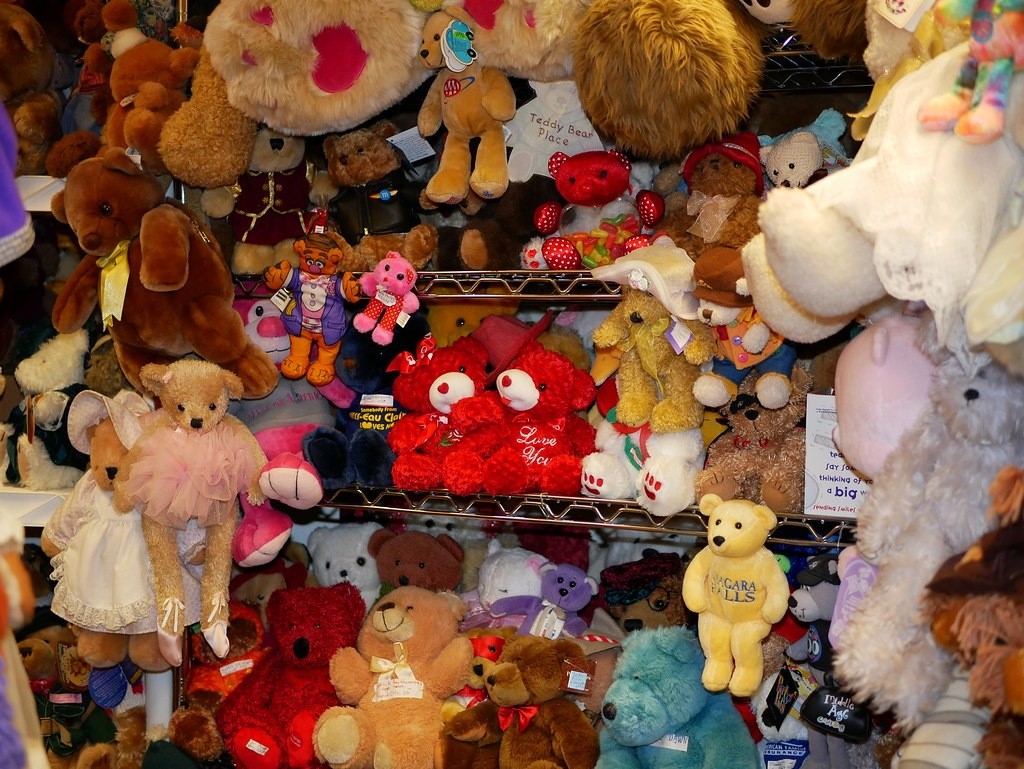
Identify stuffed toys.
[0,1,1024,768]
